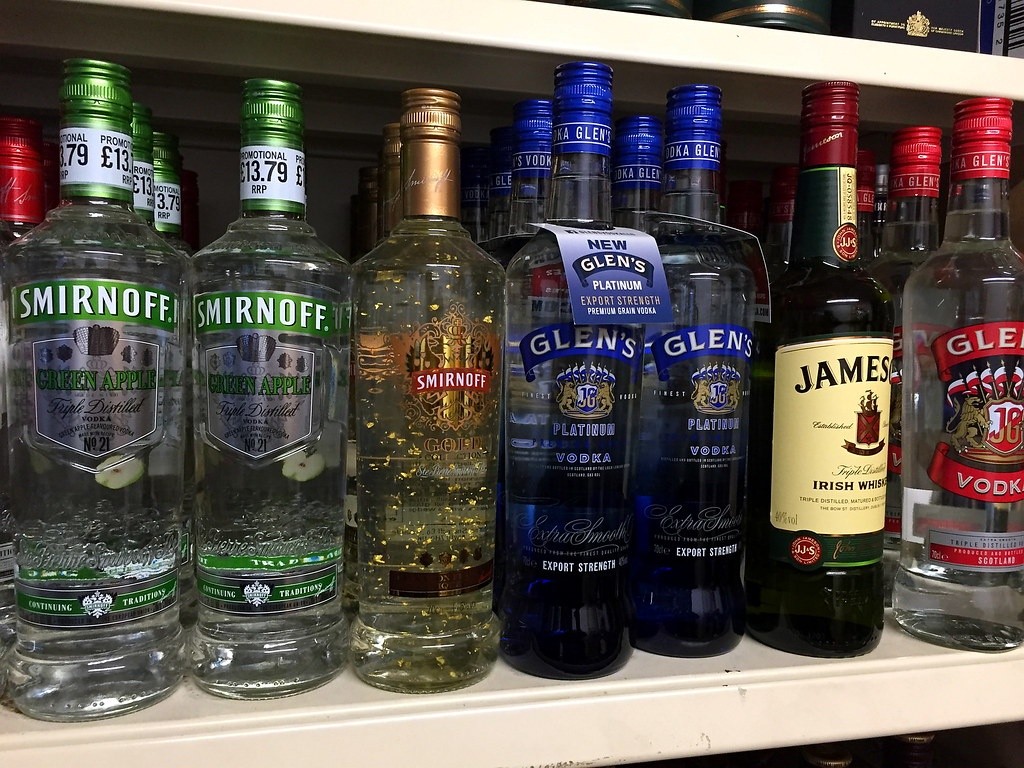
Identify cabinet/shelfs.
[0,0,1024,768]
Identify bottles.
[802,744,854,768]
[564,0,694,19]
[895,733,936,768]
[705,0,831,35]
[0,60,1024,721]
